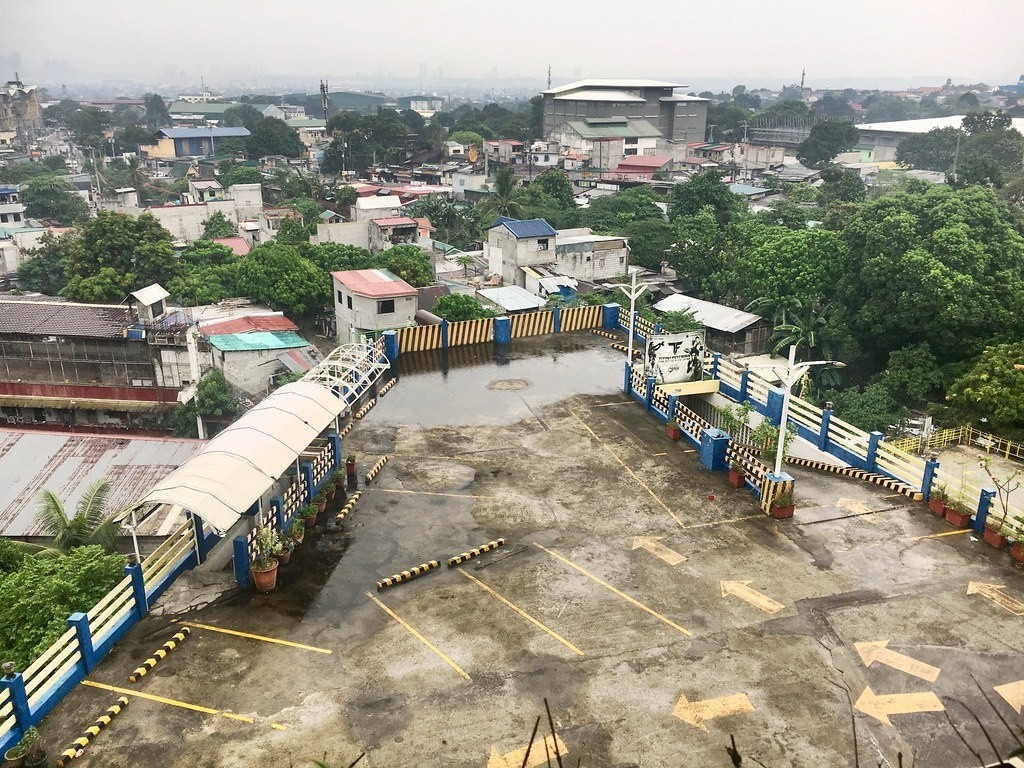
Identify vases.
[982,523,1008,550]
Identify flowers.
[976,452,1021,532]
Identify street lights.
[770,345,846,479]
[619,269,675,366]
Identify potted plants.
[4,727,49,768]
[945,498,973,529]
[345,454,358,475]
[665,417,681,440]
[771,488,796,520]
[928,485,951,517]
[729,460,746,488]
[272,468,345,564]
[248,522,283,593]
[1010,516,1024,563]
